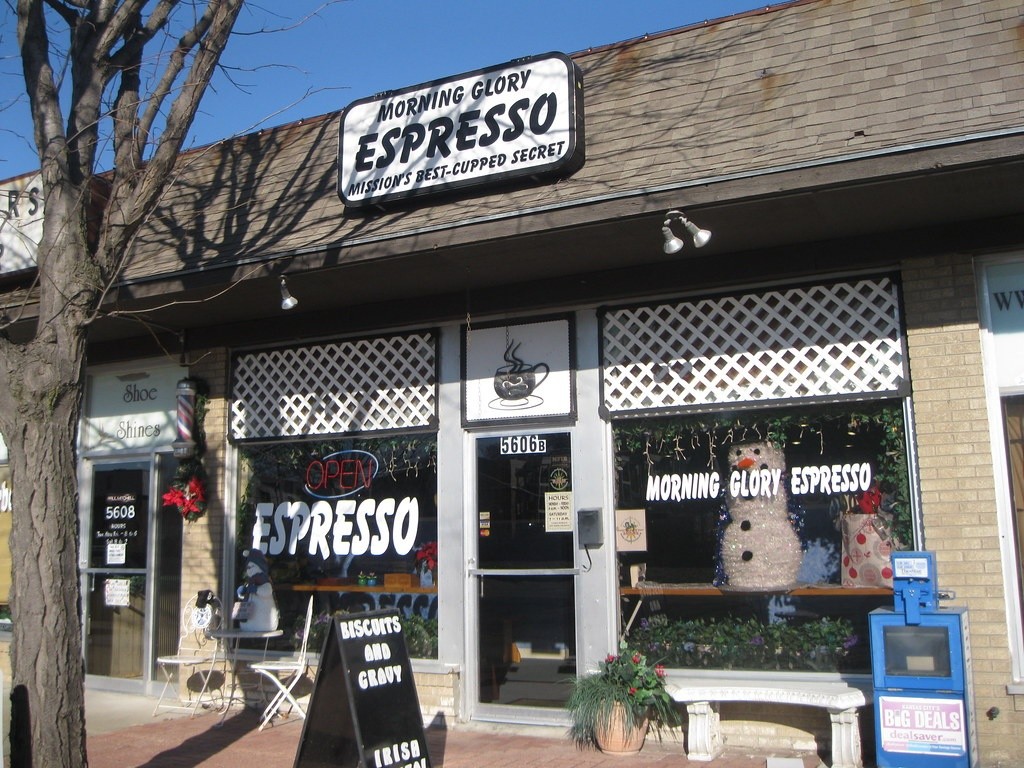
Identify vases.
[592,698,650,755]
[420,562,432,588]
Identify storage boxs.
[384,573,419,588]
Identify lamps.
[680,216,712,248]
[656,218,683,254]
[279,275,298,310]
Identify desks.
[274,584,438,596]
[206,629,284,728]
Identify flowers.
[570,647,683,752]
[413,541,437,569]
[162,476,206,517]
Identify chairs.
[153,590,225,719]
[247,595,314,731]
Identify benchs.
[662,677,875,768]
